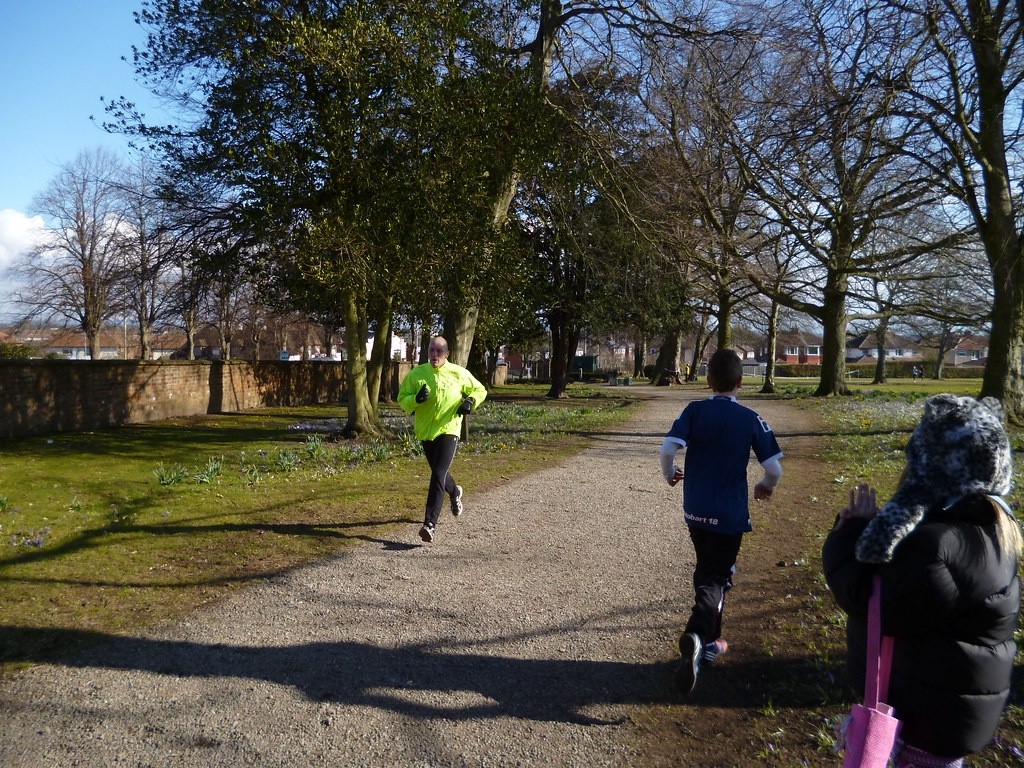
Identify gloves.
[456,397,475,418]
[415,384,429,404]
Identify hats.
[853,393,1012,563]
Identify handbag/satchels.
[830,574,903,768]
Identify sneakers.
[702,639,728,662]
[419,522,434,542]
[674,633,701,696]
[450,485,463,516]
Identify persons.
[398,336,487,544]
[823,393,1024,768]
[659,347,784,692]
